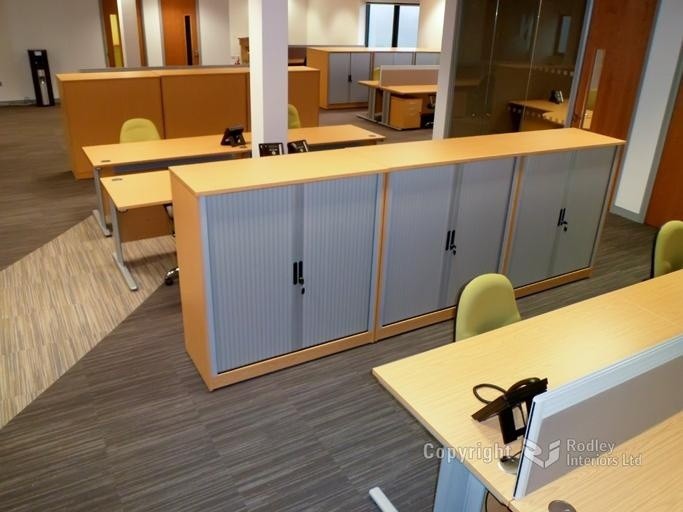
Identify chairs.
[650,216,683,277]
[120,118,163,142]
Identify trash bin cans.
[27,49,58,108]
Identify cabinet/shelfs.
[167,126,631,395]
[306,46,440,106]
[54,64,320,180]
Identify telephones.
[472,378,548,444]
[548,89,563,104]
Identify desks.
[372,269,683,511]
[82,123,385,294]
[357,62,439,135]
